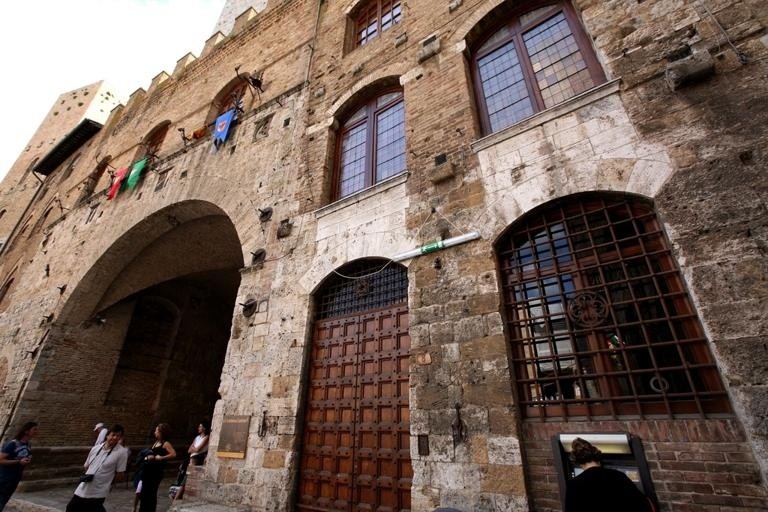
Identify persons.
[131,421,177,512]
[64,422,129,512]
[91,423,107,444]
[562,437,653,512]
[185,422,208,474]
[0,420,39,511]
[168,456,202,504]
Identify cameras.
[79,474,93,482]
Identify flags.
[104,164,132,200]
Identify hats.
[93,422,104,431]
